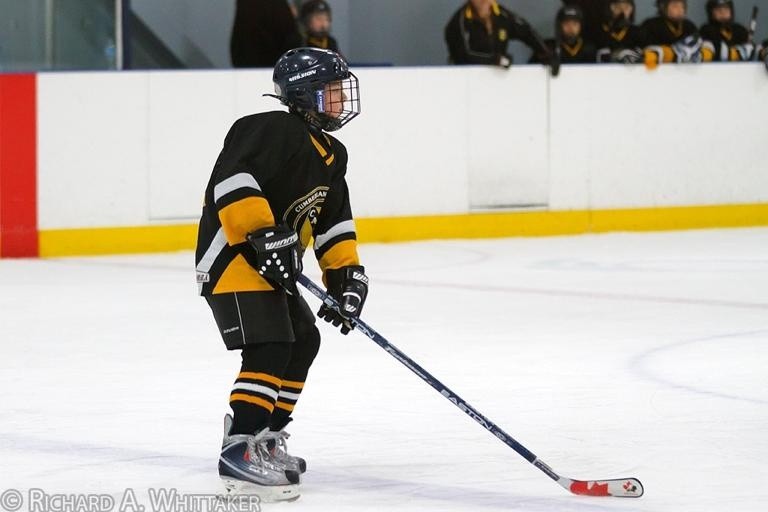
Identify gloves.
[248,223,303,298]
[318,263,371,337]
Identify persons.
[230,0,303,69]
[698,0,756,63]
[580,0,645,65]
[637,0,703,71]
[192,46,370,487]
[442,1,560,77]
[288,0,339,55]
[526,7,596,64]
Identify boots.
[219,410,309,486]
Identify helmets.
[557,0,735,43]
[273,45,352,107]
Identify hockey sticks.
[297,273,642,498]
